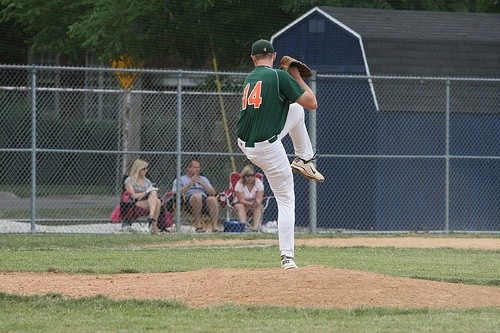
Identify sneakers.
[280,254,298,270]
[290,150,325,183]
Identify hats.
[249,39,274,61]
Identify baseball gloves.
[280,56,312,78]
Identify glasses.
[245,173,255,177]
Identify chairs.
[120,174,219,232]
[218,172,274,228]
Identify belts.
[245,135,278,148]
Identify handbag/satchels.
[163,204,174,227]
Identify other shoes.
[195,226,206,233]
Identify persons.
[124,159,162,236]
[172,159,221,232]
[235,40,324,270]
[232,165,264,233]
